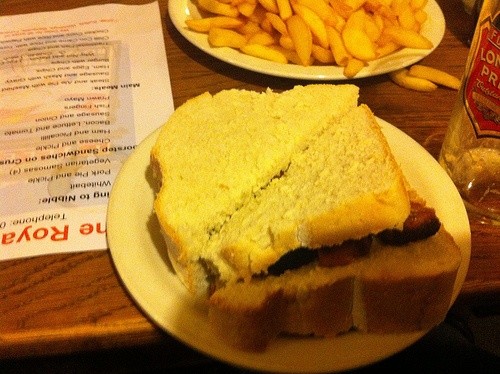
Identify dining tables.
[0,2,500,359]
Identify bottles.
[439,0,500,222]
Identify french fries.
[182,0,462,92]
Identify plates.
[106,115,471,374]
[168,0,446,80]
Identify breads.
[150,84,462,354]
